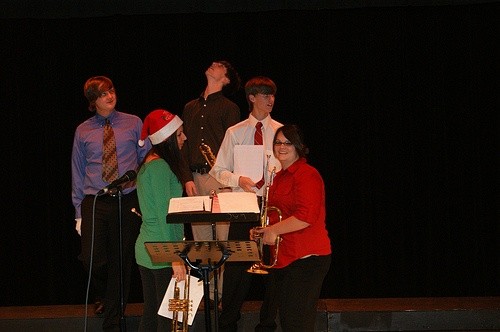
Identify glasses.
[274,139,292,147]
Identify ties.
[101,119,119,183]
[254,122,265,190]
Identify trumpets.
[131,208,186,241]
[246,186,283,274]
[168,265,192,332]
[199,143,232,192]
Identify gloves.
[75,218,82,237]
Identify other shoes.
[218,302,223,310]
[95,302,105,317]
[198,299,204,309]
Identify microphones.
[97,170,137,195]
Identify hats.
[138,109,184,147]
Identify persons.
[135,109,187,332]
[178,59,241,310]
[71,76,154,332]
[209,78,285,332]
[250,125,333,332]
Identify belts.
[102,194,119,198]
